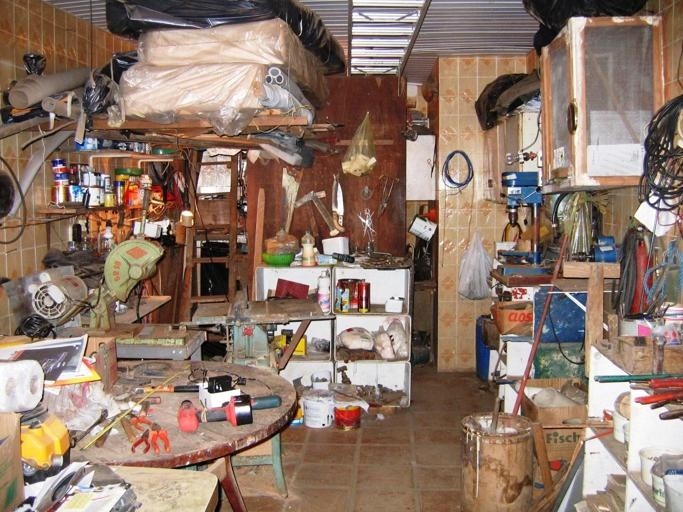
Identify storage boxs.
[0,411,29,511]
[491,297,534,335]
[514,370,584,502]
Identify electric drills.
[176,394,253,434]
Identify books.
[0,334,101,395]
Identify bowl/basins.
[261,251,296,267]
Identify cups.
[357,283,370,312]
[637,447,683,512]
[610,412,629,465]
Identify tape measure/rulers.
[79,374,178,451]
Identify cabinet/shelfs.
[581,337,682,511]
[333,264,413,409]
[481,122,505,203]
[255,267,333,415]
[497,112,542,203]
[488,266,555,436]
[542,16,665,193]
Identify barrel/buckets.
[460,413,535,512]
[287,390,364,431]
[639,447,683,512]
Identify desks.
[70,463,219,511]
[66,359,299,511]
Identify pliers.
[130,413,171,455]
[377,175,400,219]
[630,377,683,420]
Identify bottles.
[501,205,523,243]
[101,219,116,255]
[70,162,126,209]
[317,272,331,313]
[300,228,317,266]
[335,278,361,313]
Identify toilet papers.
[0,359,45,414]
